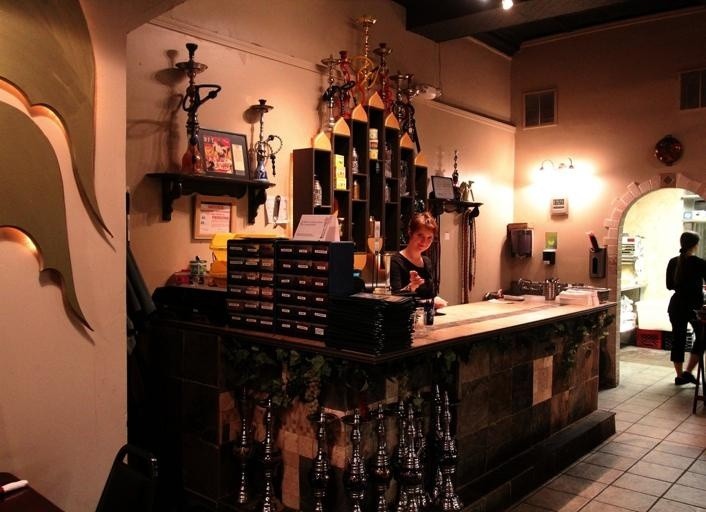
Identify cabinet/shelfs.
[293,91,428,269]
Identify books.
[337,290,416,357]
[554,289,601,307]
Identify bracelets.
[406,284,412,292]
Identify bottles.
[414,307,428,338]
[351,147,359,174]
[314,174,322,206]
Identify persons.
[664,228,706,385]
[390,212,452,313]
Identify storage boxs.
[226,237,286,333]
[275,241,356,341]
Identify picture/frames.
[192,193,238,240]
[430,176,456,200]
[198,128,251,180]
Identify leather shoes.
[681,371,700,385]
[675,377,688,385]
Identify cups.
[543,279,556,300]
